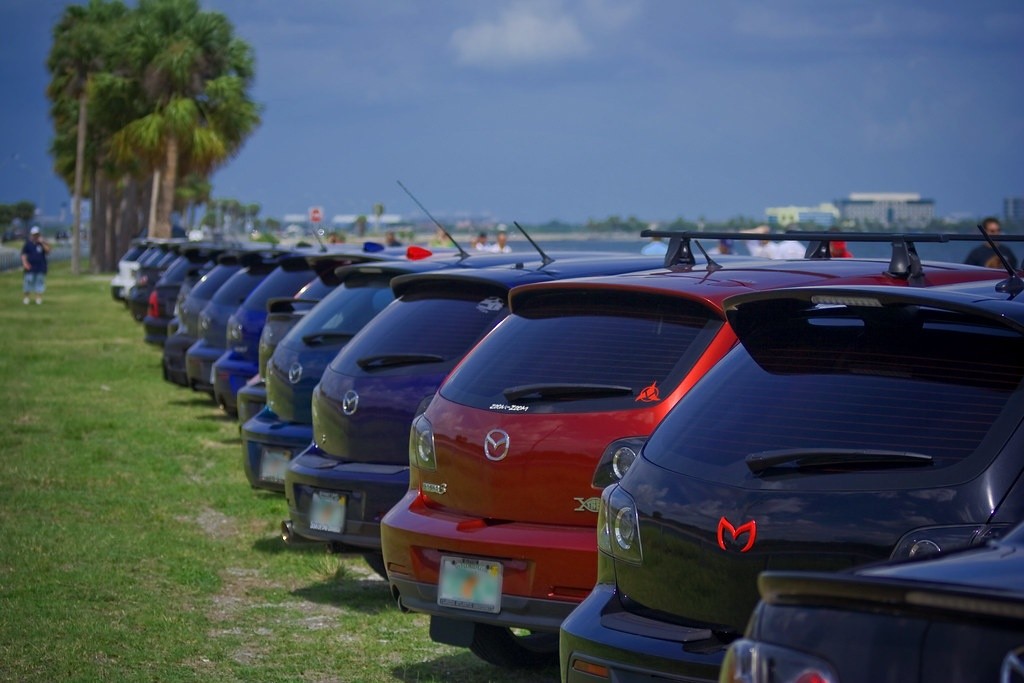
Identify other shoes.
[23,296,30,305]
[35,297,42,305]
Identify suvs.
[111,179,1024,683]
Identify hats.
[720,239,735,248]
[30,226,40,235]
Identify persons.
[708,224,853,258]
[385,231,401,246]
[428,227,456,248]
[488,224,512,253]
[21,226,49,305]
[476,231,491,251]
[964,218,1018,269]
[642,225,669,255]
